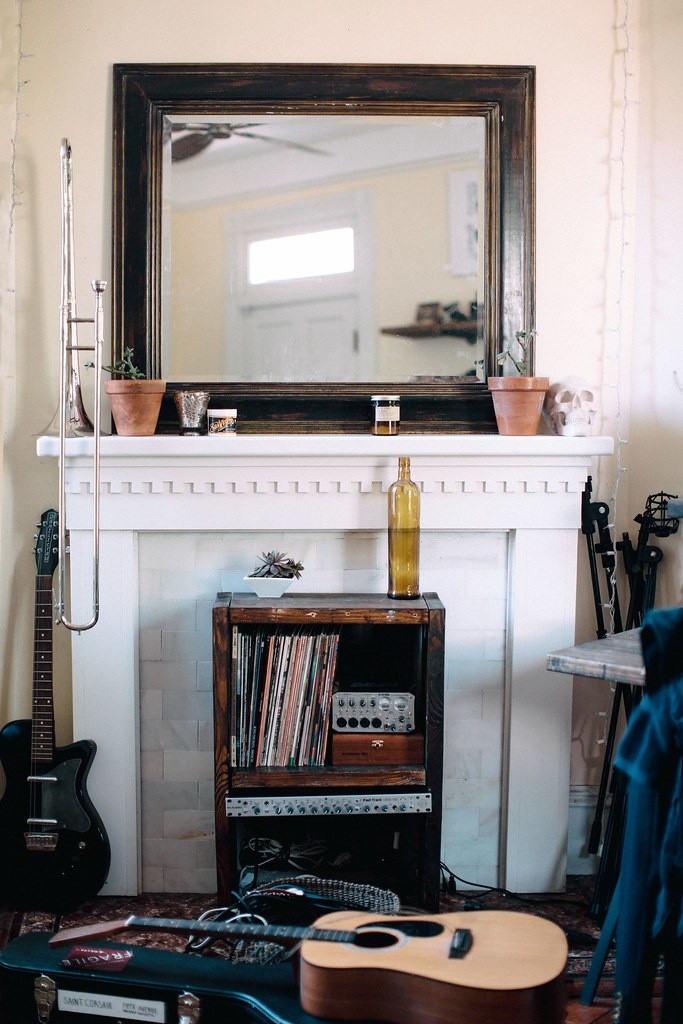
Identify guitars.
[0,509,111,911]
[46,908,571,1024]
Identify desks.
[547,626,647,686]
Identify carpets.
[7,891,668,976]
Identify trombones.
[55,135,110,633]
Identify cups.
[207,409,237,436]
[371,395,401,434]
[173,391,211,437]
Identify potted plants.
[84,347,166,437]
[487,329,548,436]
[243,552,303,599]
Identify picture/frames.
[452,165,485,275]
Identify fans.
[171,122,333,162]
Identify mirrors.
[109,63,537,434]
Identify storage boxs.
[333,733,424,764]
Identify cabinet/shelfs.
[210,593,446,909]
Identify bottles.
[388,457,421,601]
